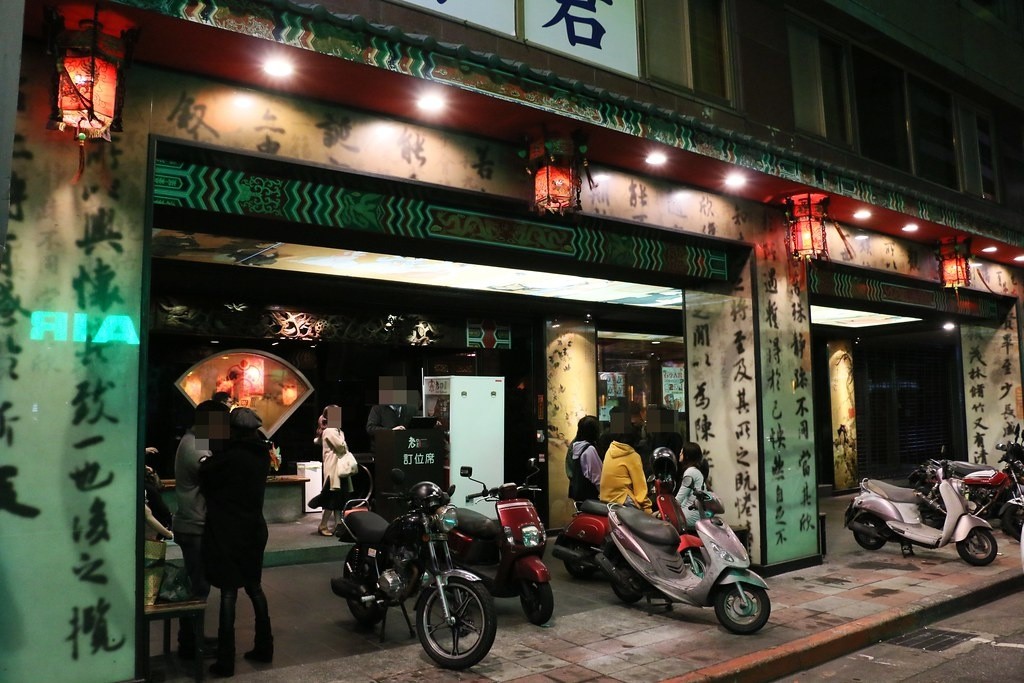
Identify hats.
[229,407,263,430]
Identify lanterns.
[44,8,137,179]
[932,236,973,289]
[512,134,593,216]
[778,186,830,284]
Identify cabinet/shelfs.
[297,463,325,513]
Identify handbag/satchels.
[161,530,200,602]
[333,443,359,478]
[143,537,167,607]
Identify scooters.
[594,473,770,635]
[844,444,998,566]
[552,446,707,581]
[429,457,555,625]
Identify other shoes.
[317,526,334,537]
[178,634,222,658]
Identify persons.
[195,406,274,677]
[308,405,353,536]
[653,442,713,525]
[565,416,603,506]
[598,425,652,513]
[172,400,230,657]
[143,447,177,546]
[366,403,412,431]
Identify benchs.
[144,600,208,665]
[158,476,310,492]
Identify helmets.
[649,447,678,473]
[409,481,446,508]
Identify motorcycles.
[907,423,1024,542]
[331,481,496,671]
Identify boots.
[245,616,275,663]
[208,629,236,677]
